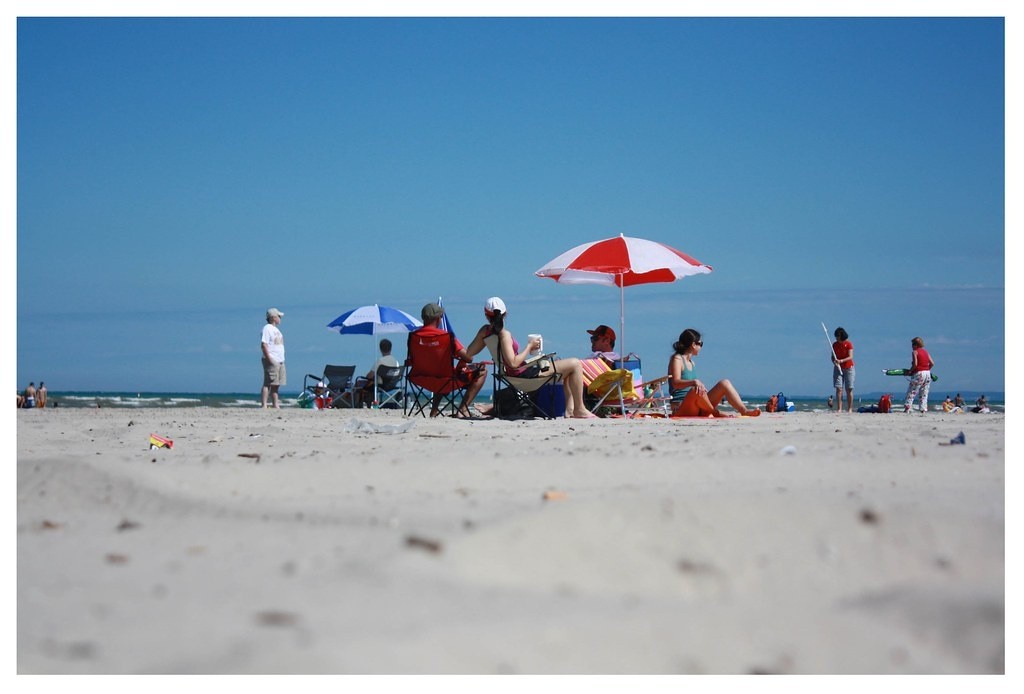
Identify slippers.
[570,414,598,418]
[460,410,494,420]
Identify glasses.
[590,336,606,342]
[695,342,703,347]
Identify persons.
[406,304,488,419]
[828,395,834,408]
[904,337,934,414]
[949,393,965,408]
[466,296,598,419]
[831,328,855,414]
[17,381,47,407]
[262,308,287,409]
[355,338,400,409]
[976,395,986,409]
[586,325,616,372]
[668,329,761,418]
[945,395,951,403]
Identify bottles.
[363,401,379,409]
[402,393,412,408]
[346,377,352,388]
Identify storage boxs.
[316,397,332,409]
[537,380,566,417]
[785,401,795,412]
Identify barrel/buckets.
[309,390,333,410]
[786,401,795,412]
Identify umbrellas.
[534,233,713,369]
[327,304,424,399]
[430,296,455,408]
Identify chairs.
[403,331,486,420]
[303,364,356,409]
[482,333,563,420]
[601,352,645,414]
[353,364,406,409]
[580,356,673,420]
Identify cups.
[528,333,542,356]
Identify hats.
[484,297,506,316]
[587,325,616,340]
[421,303,444,320]
[266,308,284,317]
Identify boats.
[718,391,794,413]
[62,395,201,408]
[219,398,299,407]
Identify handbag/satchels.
[766,392,788,412]
[297,391,317,408]
[878,394,894,412]
